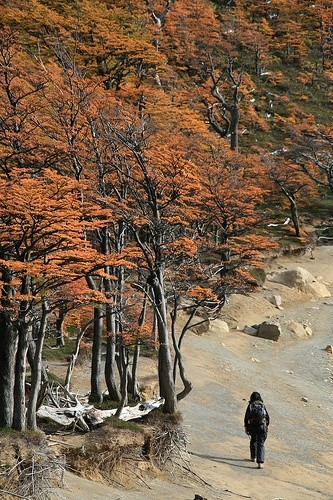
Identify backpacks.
[249,400,265,425]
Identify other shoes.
[259,464,264,469]
[251,458,255,462]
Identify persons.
[244,392,269,469]
